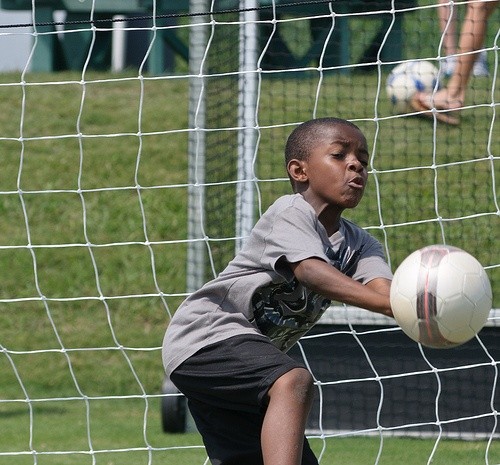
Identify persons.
[410,0,500,126]
[162,118,398,465]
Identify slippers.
[411,88,464,125]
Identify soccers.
[386,61,441,115]
[390,244,492,348]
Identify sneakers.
[475,50,488,76]
[445,57,458,76]
